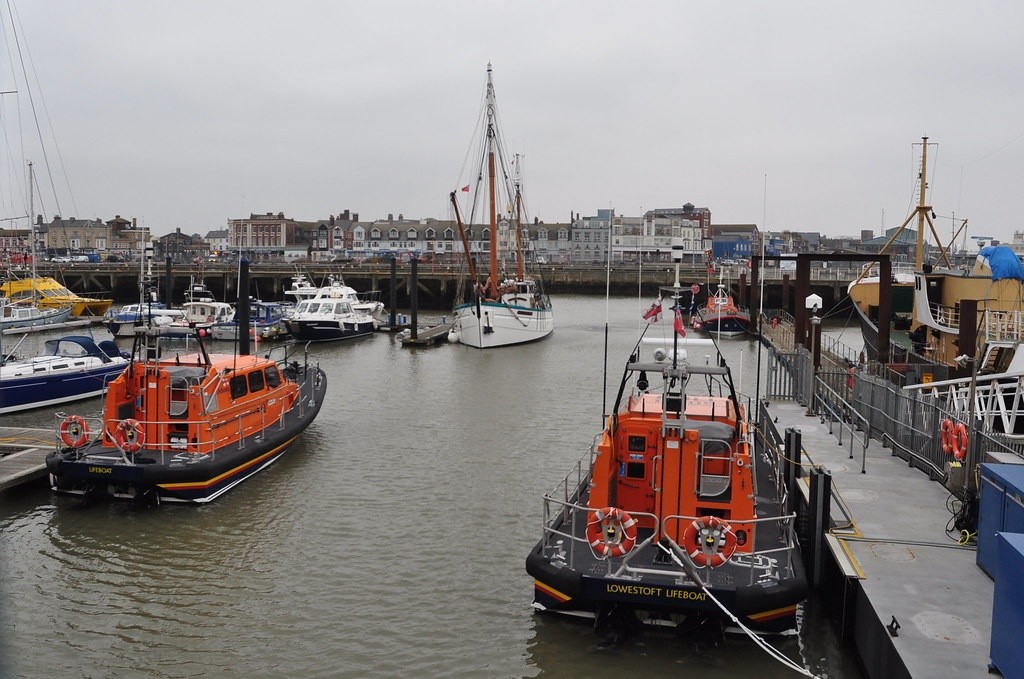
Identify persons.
[474,268,506,299]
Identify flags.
[462,185,470,192]
[641,296,662,323]
[674,308,686,338]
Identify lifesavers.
[684,516,738,567]
[941,419,953,455]
[115,419,145,452]
[586,507,637,557]
[60,415,89,447]
[952,423,968,459]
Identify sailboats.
[446,64,554,350]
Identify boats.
[46,211,328,505]
[0,335,131,415]
[690,258,754,336]
[848,138,1023,389]
[527,238,809,653]
[0,187,385,339]
